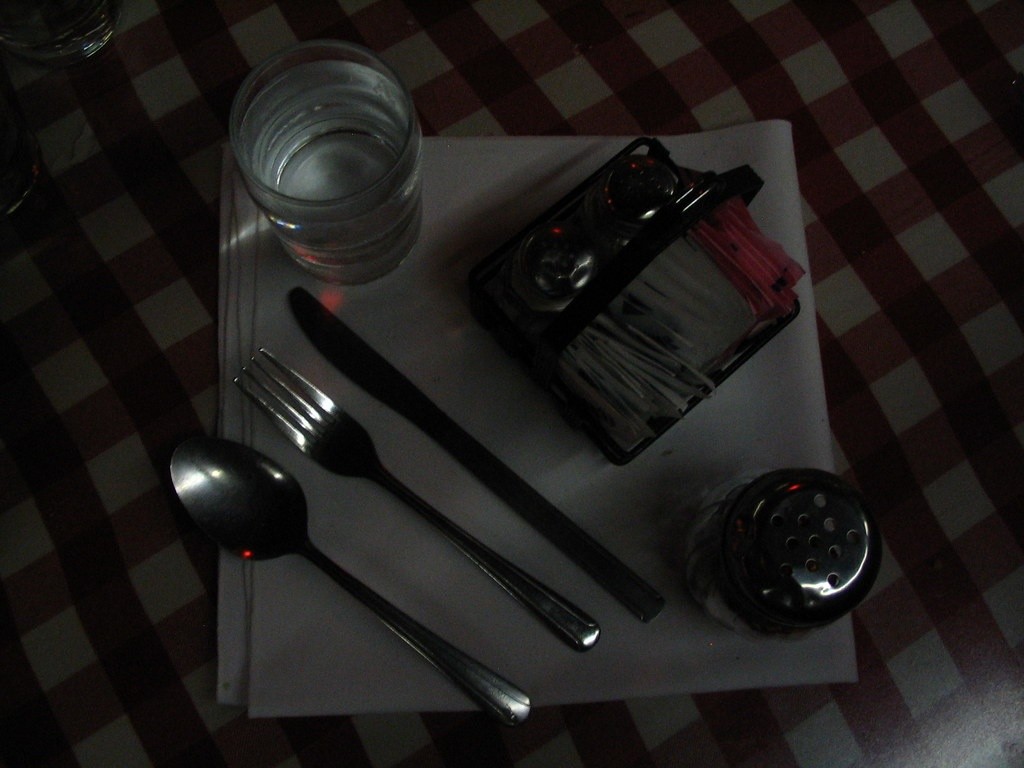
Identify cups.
[229,40,424,287]
[0,0,120,68]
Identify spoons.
[169,437,531,728]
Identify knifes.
[286,286,668,624]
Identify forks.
[232,347,602,651]
